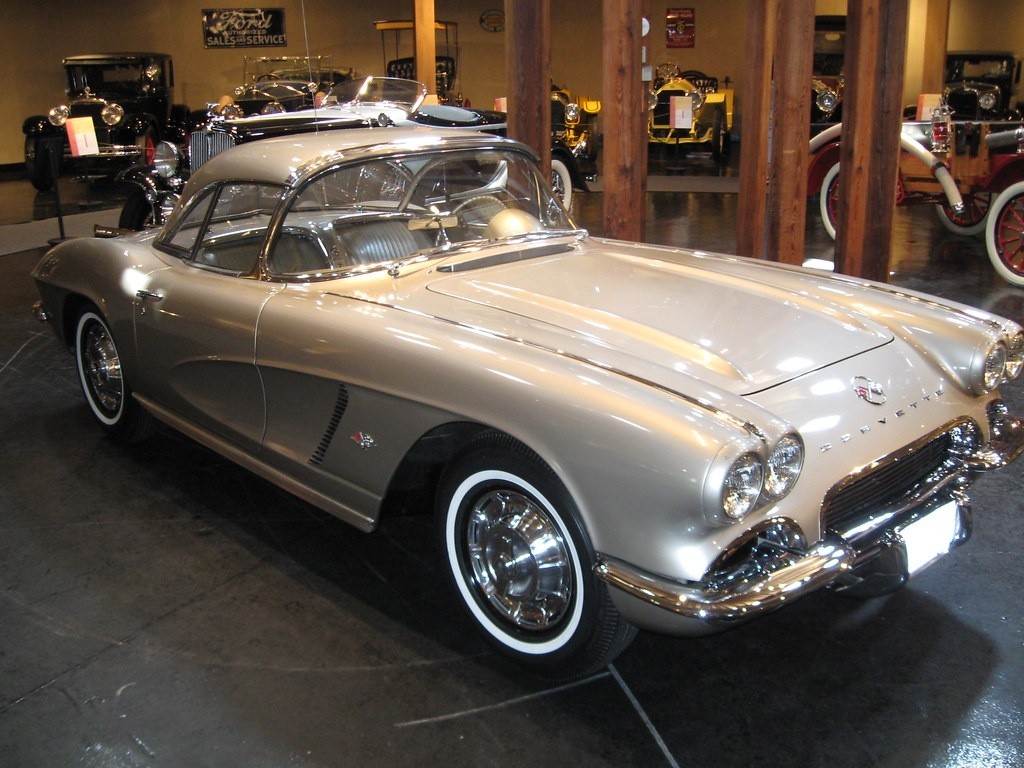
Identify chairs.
[195,225,335,272]
[332,211,434,264]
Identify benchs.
[271,71,346,83]
[653,77,718,95]
[388,55,455,91]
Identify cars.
[115,76,505,230]
[548,76,601,161]
[810,14,847,123]
[647,63,735,156]
[809,104,1024,288]
[904,49,1024,156]
[22,52,190,192]
[191,55,377,133]
[29,126,1024,679]
